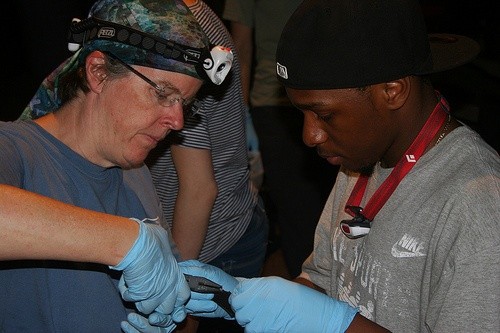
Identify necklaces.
[435,114,452,146]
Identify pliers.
[110,268,222,294]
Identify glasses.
[101,50,201,116]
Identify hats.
[276,0,481,90]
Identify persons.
[177,0,499,333]
[0,0,341,333]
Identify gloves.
[121,307,187,333]
[178,259,239,320]
[108,218,191,315]
[229,276,359,332]
